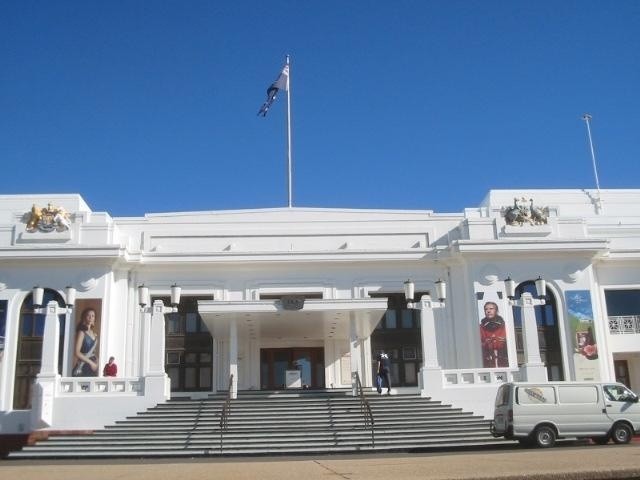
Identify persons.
[103,357,117,376]
[72,307,99,377]
[479,302,509,367]
[376,350,391,395]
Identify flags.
[257,63,288,117]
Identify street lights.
[32,282,76,378]
[403,279,446,373]
[504,276,544,367]
[138,283,181,377]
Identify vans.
[490,383,640,449]
